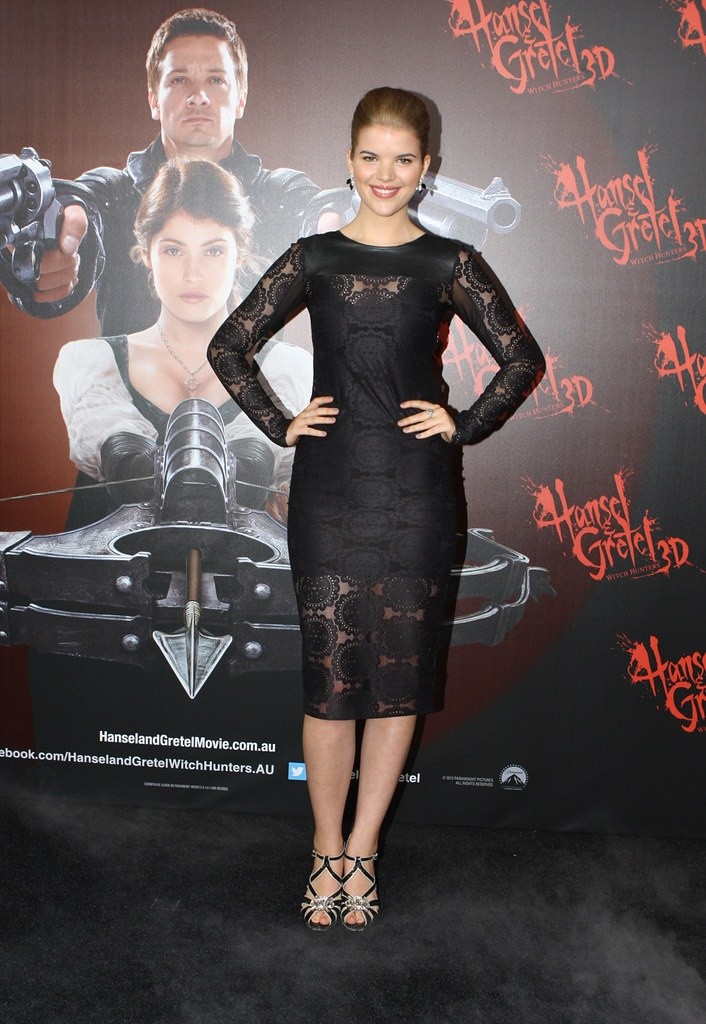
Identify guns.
[0,146,75,312]
[342,170,522,252]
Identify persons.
[23,5,352,523]
[207,85,548,933]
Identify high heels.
[340,843,381,932]
[300,849,343,930]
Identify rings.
[426,408,434,417]
[305,410,311,418]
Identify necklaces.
[153,321,211,395]
